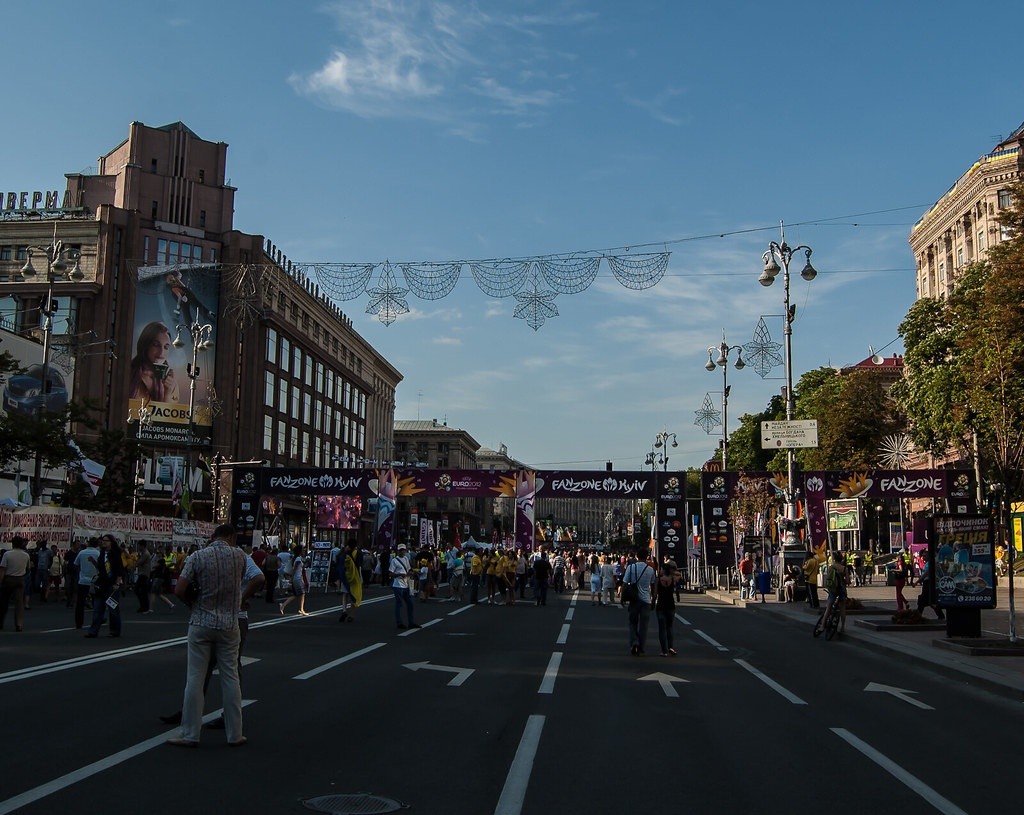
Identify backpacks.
[825,566,845,592]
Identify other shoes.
[279,603,284,616]
[206,713,226,729]
[906,604,909,609]
[631,646,641,655]
[159,710,182,724]
[338,612,348,624]
[14,596,176,637]
[166,738,200,748]
[659,651,669,657]
[408,623,422,628]
[417,585,617,607]
[348,617,354,622]
[669,648,677,655]
[299,610,309,616]
[396,623,410,630]
[229,736,247,745]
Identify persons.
[817,550,850,632]
[514,544,586,607]
[329,541,400,624]
[994,537,1018,577]
[845,549,874,587]
[391,541,518,630]
[784,563,797,603]
[158,524,291,749]
[802,551,821,608]
[278,545,309,616]
[888,547,946,621]
[738,551,760,602]
[0,536,63,632]
[587,548,681,658]
[128,322,180,403]
[64,534,175,638]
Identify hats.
[592,555,599,563]
[397,543,407,550]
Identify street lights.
[19,239,84,506]
[705,345,746,469]
[645,452,664,471]
[172,323,215,518]
[655,432,678,471]
[755,240,818,545]
[127,408,154,514]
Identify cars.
[3,364,68,418]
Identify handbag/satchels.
[893,561,908,579]
[408,579,414,596]
[106,588,120,610]
[804,575,810,581]
[622,582,639,602]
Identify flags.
[64,440,107,498]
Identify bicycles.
[813,589,848,641]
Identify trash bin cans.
[758,571,771,594]
[885,570,902,586]
